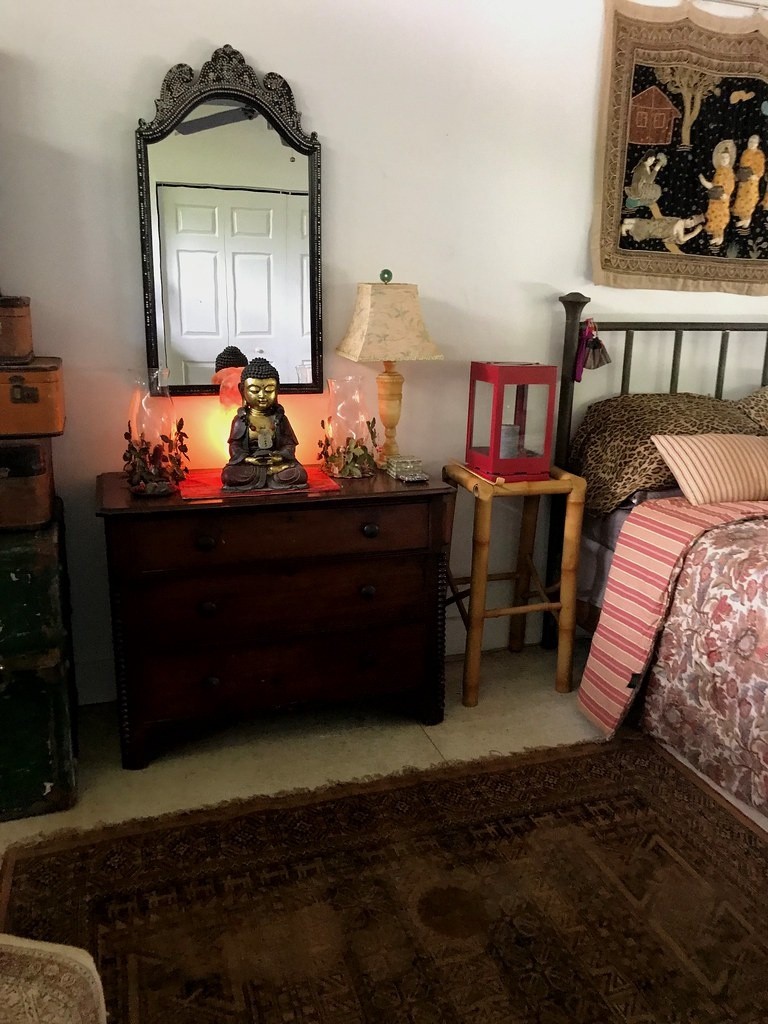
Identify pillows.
[572,391,768,520]
[651,432,768,504]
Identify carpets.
[0,733,768,1024]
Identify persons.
[213,346,252,373]
[221,358,308,486]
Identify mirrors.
[136,44,322,396]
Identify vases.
[319,371,384,480]
[122,374,191,495]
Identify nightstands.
[444,465,587,705]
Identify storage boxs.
[0,438,56,532]
[0,356,66,439]
[0,294,34,366]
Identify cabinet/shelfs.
[0,496,79,825]
[100,461,457,770]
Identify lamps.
[337,269,444,470]
[464,358,556,482]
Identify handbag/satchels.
[576,321,611,381]
